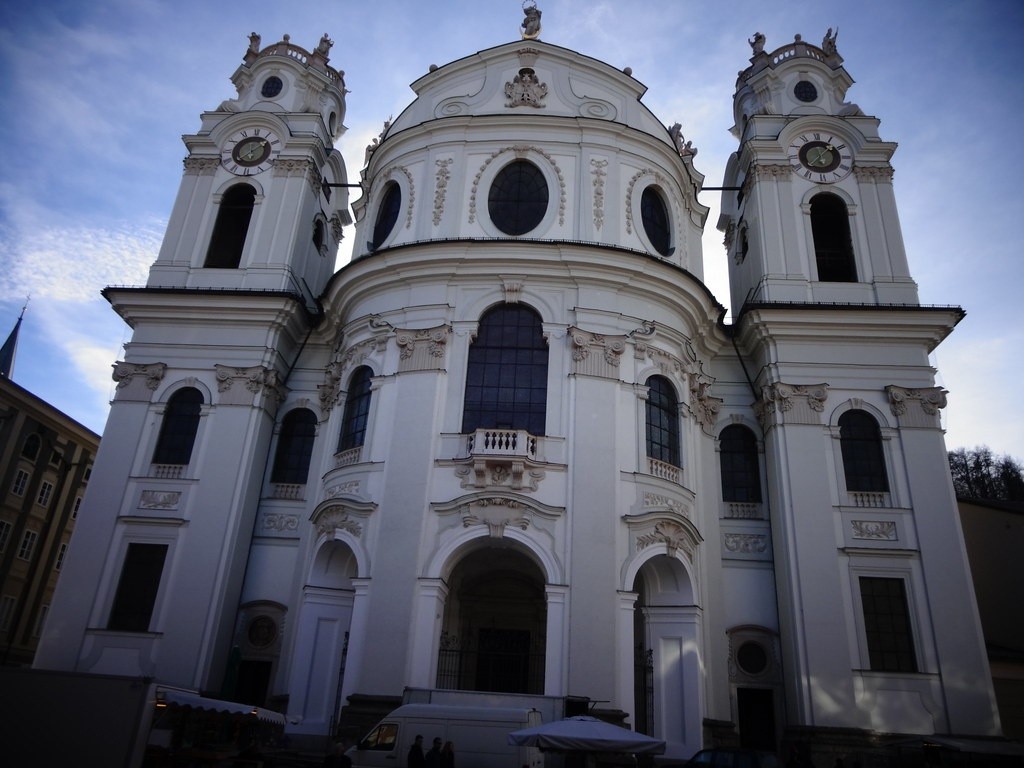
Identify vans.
[684,748,781,768]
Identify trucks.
[343,704,543,768]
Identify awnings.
[165,691,287,725]
[507,716,666,755]
[925,736,1024,755]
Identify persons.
[407,735,455,768]
[324,742,351,768]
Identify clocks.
[220,127,280,176]
[789,129,854,183]
